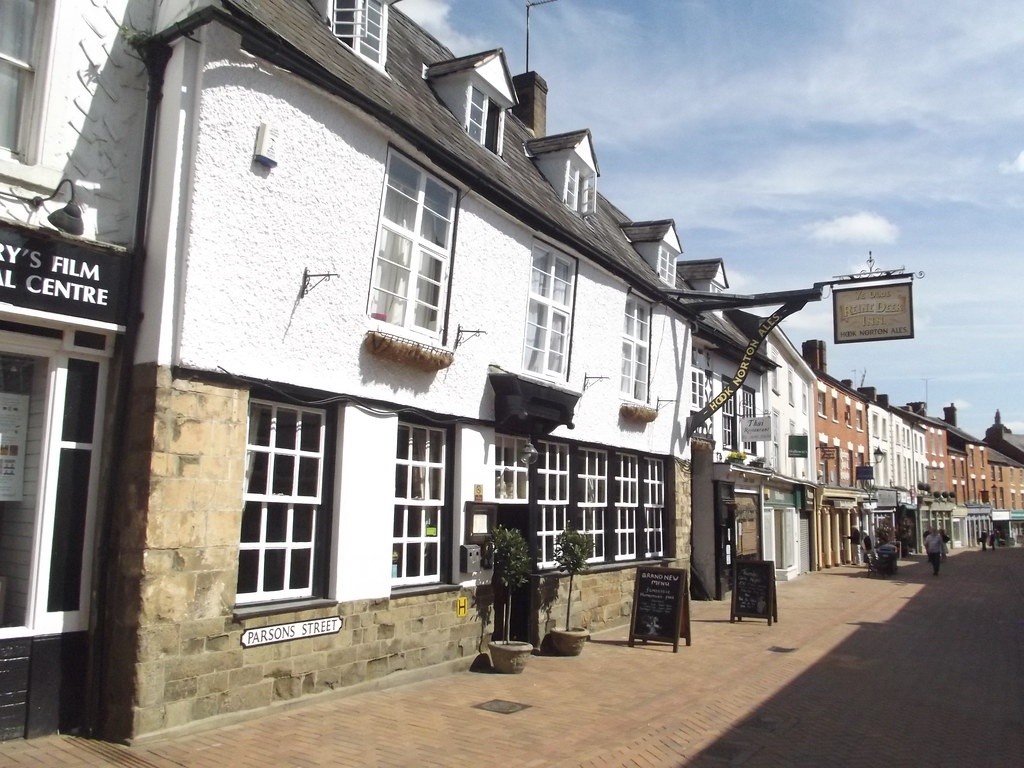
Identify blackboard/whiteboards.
[732,561,773,618]
[633,566,687,637]
[890,541,901,558]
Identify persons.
[861,530,872,568]
[977,528,1001,551]
[922,526,950,576]
[842,525,861,566]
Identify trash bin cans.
[877,546,897,575]
